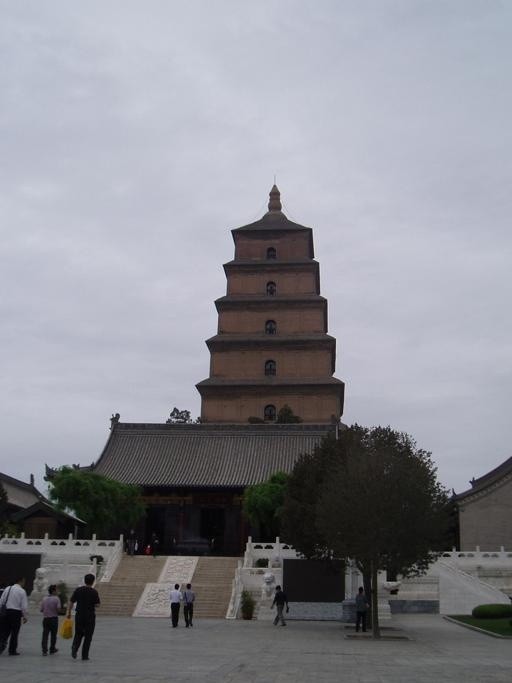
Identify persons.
[270,584,289,627]
[125,526,159,559]
[354,586,370,632]
[67,573,100,661]
[39,582,66,656]
[183,584,196,627]
[169,584,182,628]
[0,573,28,656]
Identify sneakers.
[42,648,58,655]
[72,645,89,660]
[9,651,21,655]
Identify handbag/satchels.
[0,606,9,625]
